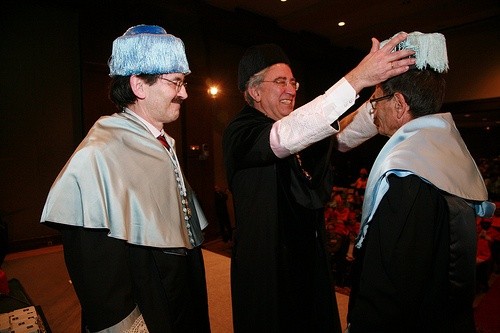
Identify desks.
[0,278,53,333]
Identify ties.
[158,135,170,149]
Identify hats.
[108,25,191,77]
[380,31,449,75]
[238,44,290,89]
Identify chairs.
[323,186,500,297]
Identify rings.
[390,61,394,69]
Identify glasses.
[158,77,187,92]
[370,94,393,109]
[258,79,299,91]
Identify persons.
[345,30,489,333]
[223,30,419,333]
[39,21,212,333]
